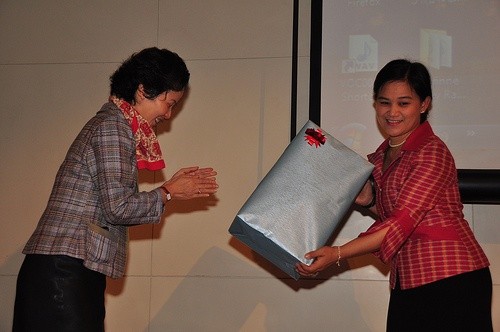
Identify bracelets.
[159,186,171,202]
[335,246,342,268]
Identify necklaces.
[388,140,405,148]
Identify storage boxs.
[226,120,376,282]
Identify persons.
[12,46,220,332]
[294,59,494,332]
